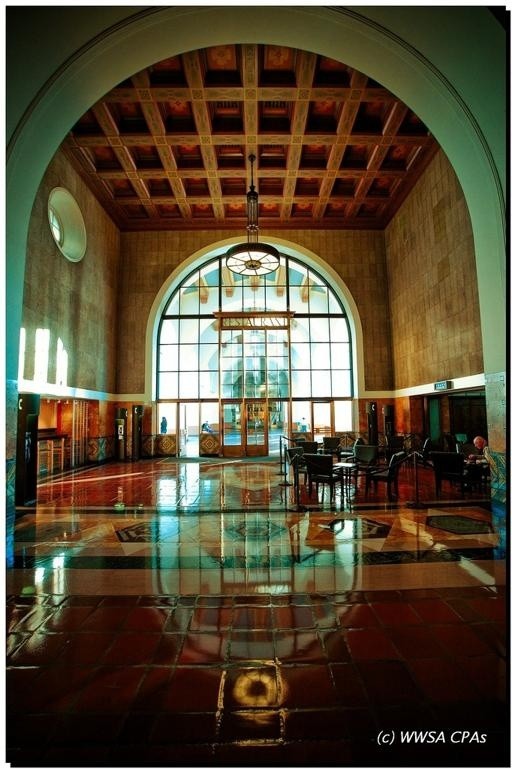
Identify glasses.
[226,154,280,276]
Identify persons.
[203,421,210,432]
[299,417,308,432]
[208,426,214,432]
[160,416,168,434]
[456,435,490,492]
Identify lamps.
[288,435,489,504]
